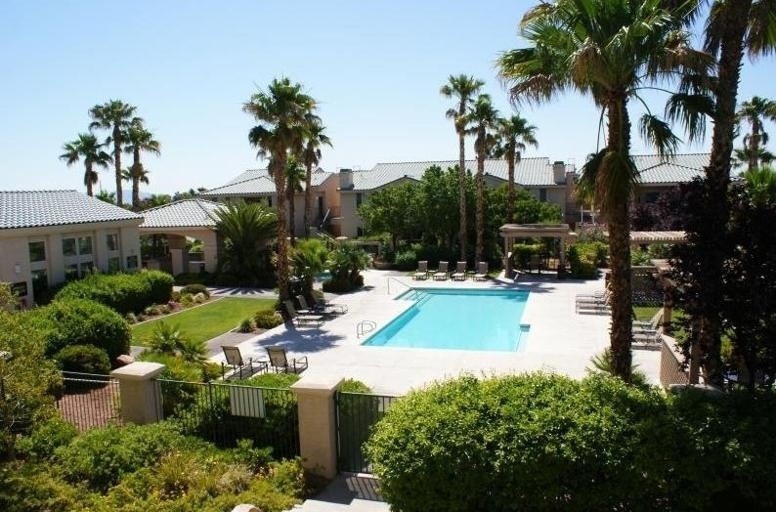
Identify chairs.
[575,283,613,315]
[282,290,348,327]
[608,308,664,349]
[412,261,488,282]
[221,346,308,380]
